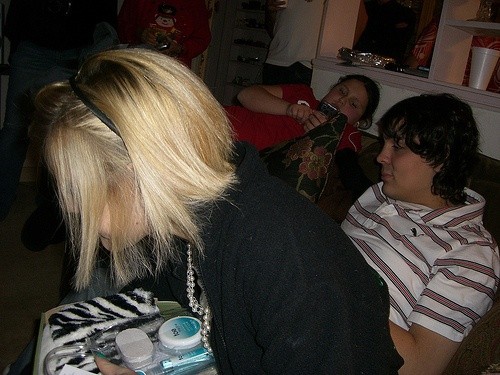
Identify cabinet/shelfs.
[211,0,272,105]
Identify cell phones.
[316,100,340,119]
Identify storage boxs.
[32,301,216,375]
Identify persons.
[32,48,406,375]
[219,75,380,153]
[116,0,213,71]
[12,92,500,375]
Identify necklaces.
[181,238,212,322]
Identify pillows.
[441,287,500,375]
[258,113,349,205]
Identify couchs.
[335,132,500,250]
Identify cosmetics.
[113,316,217,375]
